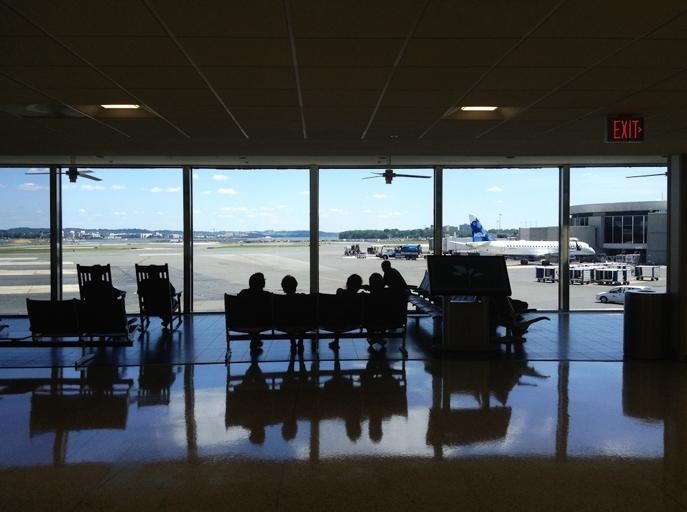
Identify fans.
[25,151,103,183]
[363,136,431,184]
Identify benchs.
[223,292,409,363]
[26,297,138,341]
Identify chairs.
[77,263,183,334]
[407,270,550,357]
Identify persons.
[138,326,177,400]
[327,273,363,347]
[361,346,393,442]
[80,264,127,302]
[280,349,309,440]
[236,272,273,349]
[281,275,306,353]
[136,265,177,327]
[327,346,361,441]
[380,261,411,297]
[362,271,401,347]
[85,334,121,395]
[236,350,268,443]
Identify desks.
[624,289,667,360]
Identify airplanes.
[448,217,596,266]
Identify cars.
[596,285,656,304]
